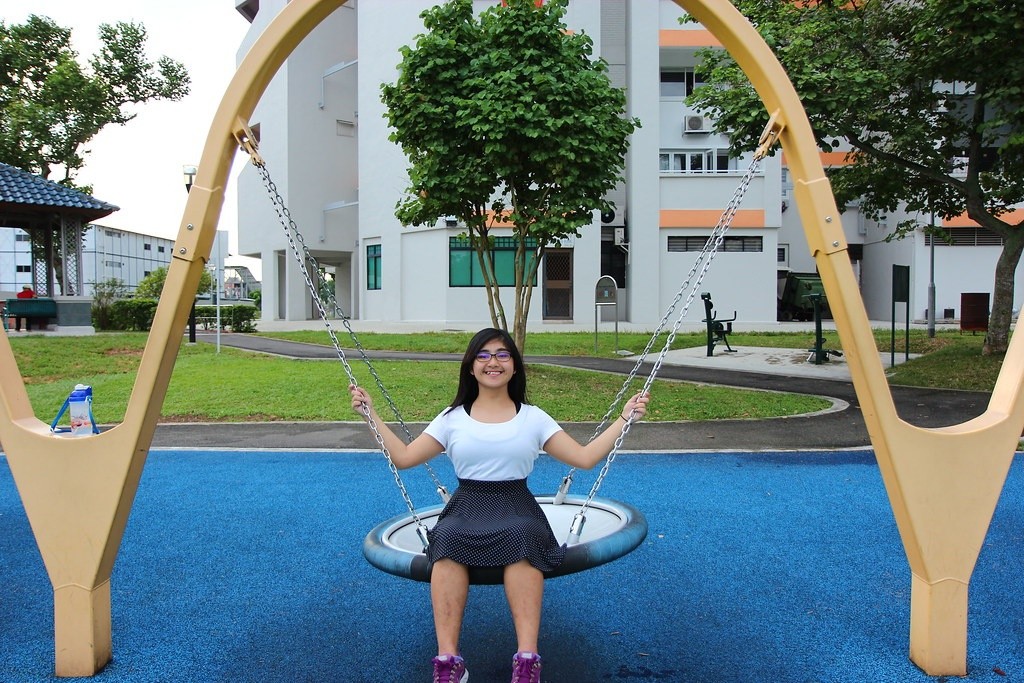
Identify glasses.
[474,351,512,362]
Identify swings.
[246,133,778,583]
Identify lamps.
[446,218,458,226]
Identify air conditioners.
[601,206,626,227]
[684,115,710,133]
[615,228,625,246]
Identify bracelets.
[620,414,632,425]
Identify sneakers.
[432,655,469,683]
[511,652,541,683]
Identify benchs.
[1,299,57,332]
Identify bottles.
[68,383,93,438]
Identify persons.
[348,328,650,683]
[15,285,38,331]
